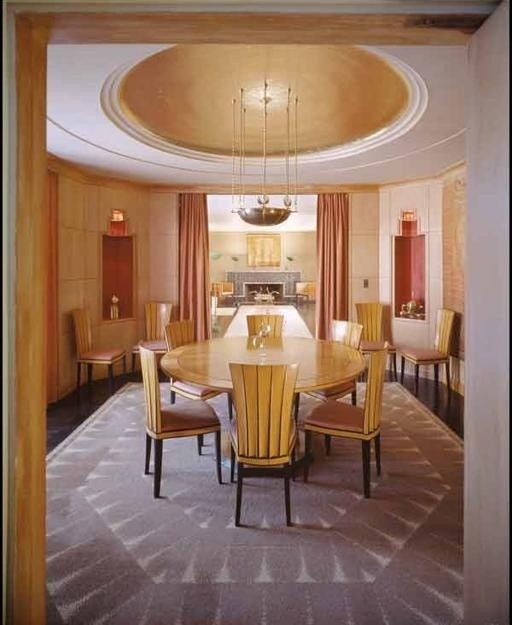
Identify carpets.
[45,383,465,625]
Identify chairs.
[246,314,284,338]
[307,319,364,406]
[226,362,299,527]
[132,301,173,381]
[138,344,223,498]
[214,281,235,302]
[399,308,457,397]
[302,350,387,499]
[70,306,128,398]
[163,319,233,447]
[353,302,398,383]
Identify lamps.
[231,79,299,225]
